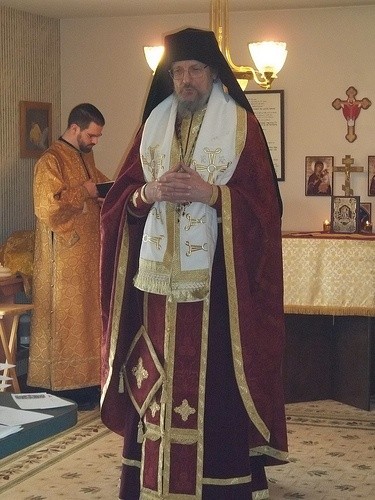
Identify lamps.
[144,0,288,92]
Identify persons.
[29,121,47,150]
[27,103,113,411]
[100,27,291,500]
[307,160,332,196]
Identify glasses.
[169,65,208,80]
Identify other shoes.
[58,388,99,410]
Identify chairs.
[2,230,35,279]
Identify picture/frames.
[368,156,375,196]
[304,156,332,194]
[332,197,360,232]
[19,100,52,158]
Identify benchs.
[0,303,34,392]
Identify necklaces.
[176,110,204,222]
